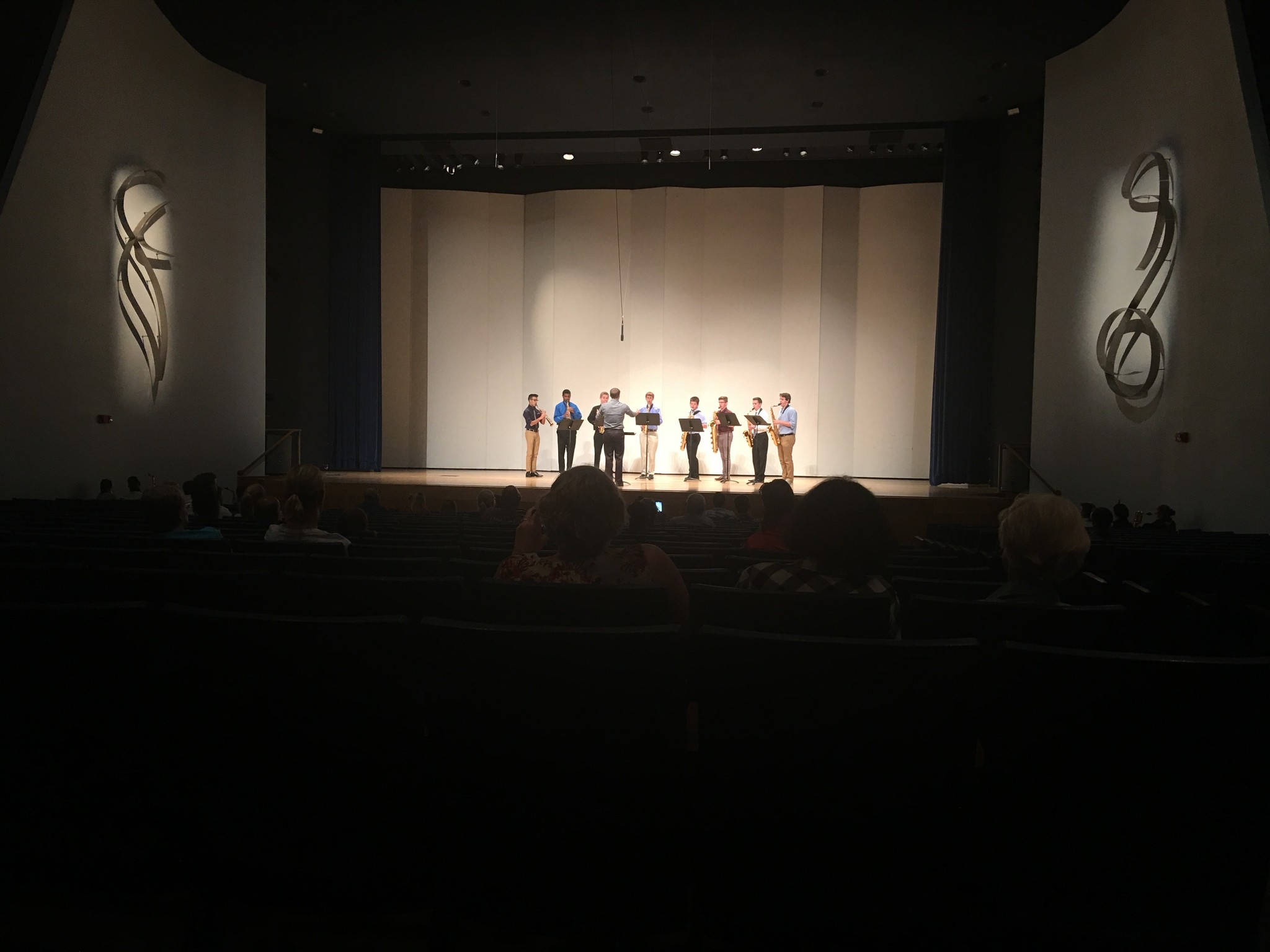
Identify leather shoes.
[530,472,543,477]
[525,472,533,477]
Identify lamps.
[799,147,807,156]
[562,153,574,160]
[782,148,791,157]
[720,149,728,160]
[384,153,522,176]
[656,151,664,163]
[640,151,649,164]
[845,142,944,156]
[670,150,681,156]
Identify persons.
[1079,501,1178,539]
[737,476,902,640]
[979,494,1091,607]
[97,470,285,540]
[264,465,351,556]
[403,492,431,515]
[358,486,387,514]
[338,506,367,535]
[771,393,797,485]
[748,397,769,483]
[474,486,550,525]
[684,397,702,481]
[494,465,688,624]
[587,392,609,469]
[439,499,457,517]
[595,388,633,486]
[627,479,797,553]
[640,392,663,480]
[554,390,582,475]
[523,394,546,478]
[713,396,734,482]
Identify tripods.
[743,415,771,485]
[634,413,660,480]
[678,418,704,481]
[716,413,742,483]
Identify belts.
[779,433,793,437]
[526,428,538,432]
[642,428,657,432]
[718,431,732,435]
[605,428,622,430]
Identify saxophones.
[743,408,755,448]
[642,401,650,434]
[709,406,721,453]
[598,401,606,434]
[680,407,694,451]
[567,400,572,419]
[536,404,554,427]
[766,403,782,446]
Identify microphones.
[621,318,624,341]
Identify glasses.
[718,402,726,404]
[759,482,765,500]
[531,399,538,401]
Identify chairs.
[0,485,1270,758]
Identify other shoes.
[616,482,623,486]
[748,479,764,483]
[714,477,725,482]
[648,474,654,479]
[639,475,646,478]
[785,479,793,484]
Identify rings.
[523,518,528,521]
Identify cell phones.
[655,500,663,513]
